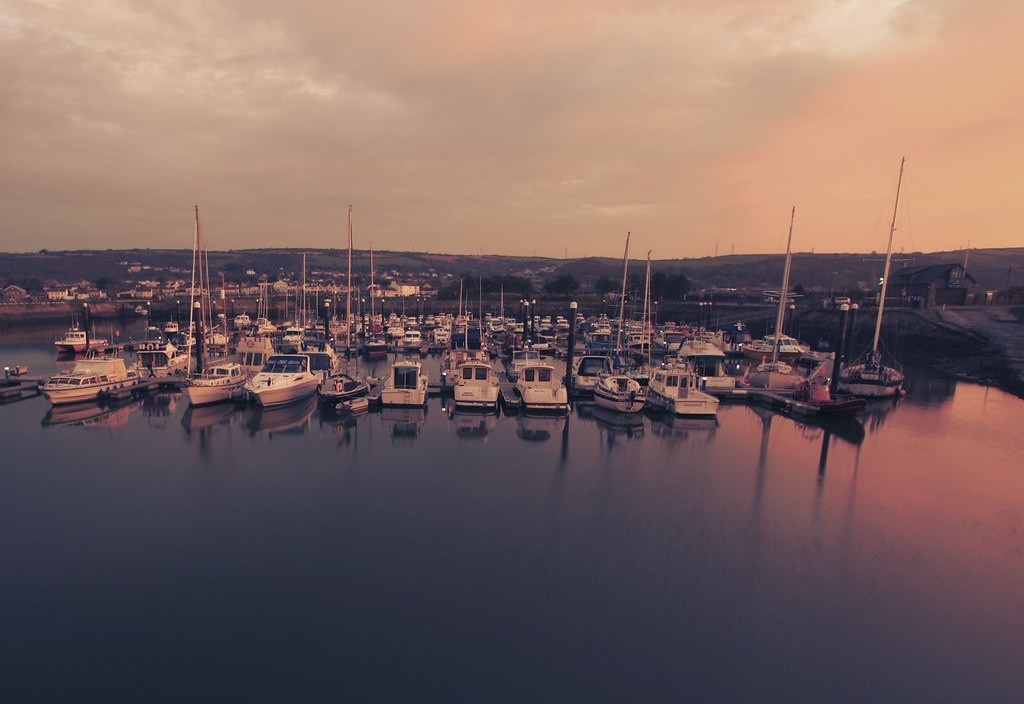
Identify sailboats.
[837,154,904,397]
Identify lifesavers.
[336,383,342,392]
[239,334,246,343]
[247,340,255,348]
[254,336,261,342]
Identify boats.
[380,404,429,446]
[516,407,570,442]
[245,392,319,442]
[446,407,504,437]
[40,393,146,435]
[592,403,647,452]
[38,198,837,417]
[181,399,238,449]
[651,407,720,436]
